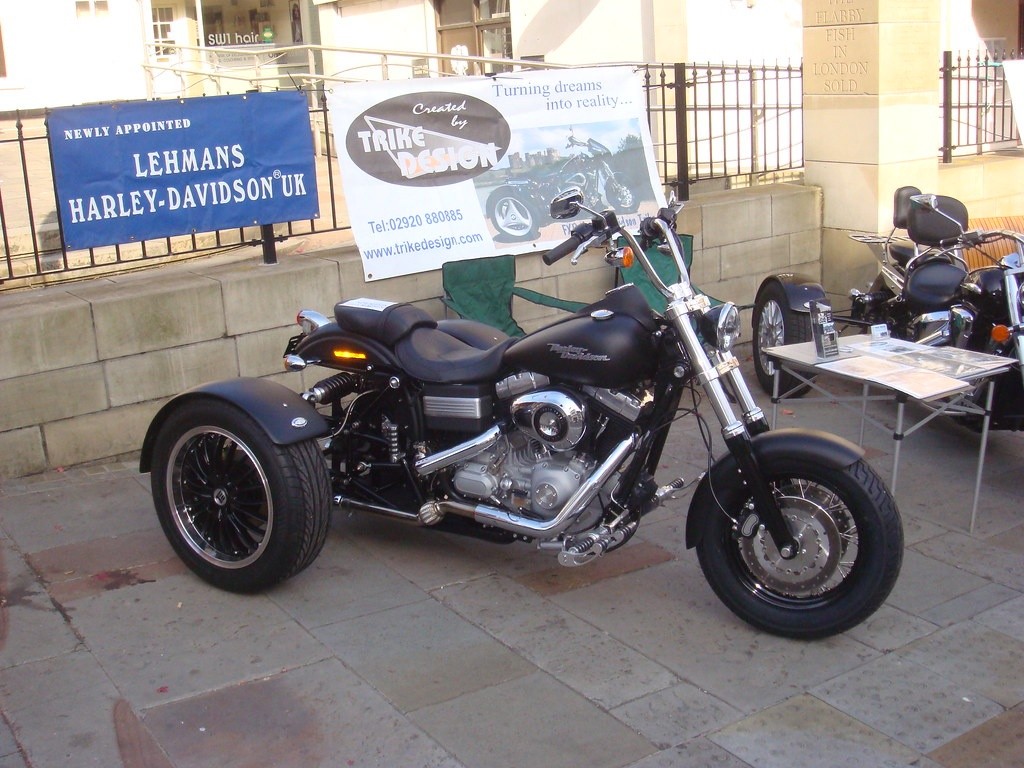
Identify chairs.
[615,234,755,404]
[440,255,591,338]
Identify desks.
[766,333,1019,534]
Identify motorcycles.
[136,186,908,642]
[753,183,1024,432]
[486,124,644,243]
